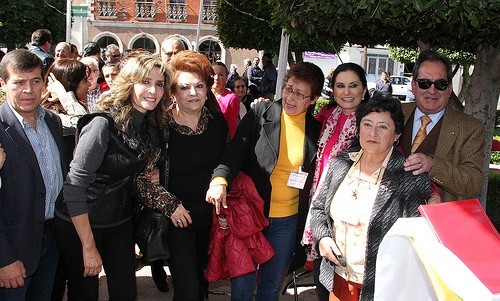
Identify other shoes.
[154,279,170,293]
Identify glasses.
[112,53,121,58]
[281,84,311,100]
[416,78,449,91]
[336,255,359,281]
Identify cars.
[367,75,410,101]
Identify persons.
[311,98,432,301]
[212,53,276,139]
[300,63,371,301]
[40,58,91,301]
[0,49,72,301]
[0,29,185,114]
[376,72,392,98]
[137,50,230,301]
[321,68,335,100]
[63,54,173,301]
[205,62,323,301]
[398,50,486,203]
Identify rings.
[177,221,180,224]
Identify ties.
[410,116,432,155]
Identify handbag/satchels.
[132,201,173,261]
